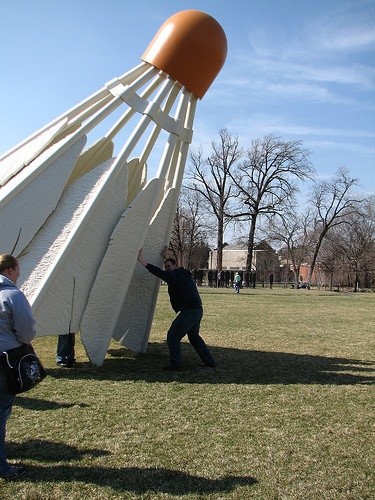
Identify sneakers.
[0,466,28,479]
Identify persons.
[138,247,215,372]
[217,271,222,288]
[234,272,241,294]
[57,333,81,367]
[0,254,36,480]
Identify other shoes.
[55,361,81,366]
[161,365,179,371]
[199,362,217,368]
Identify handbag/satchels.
[0,343,47,395]
